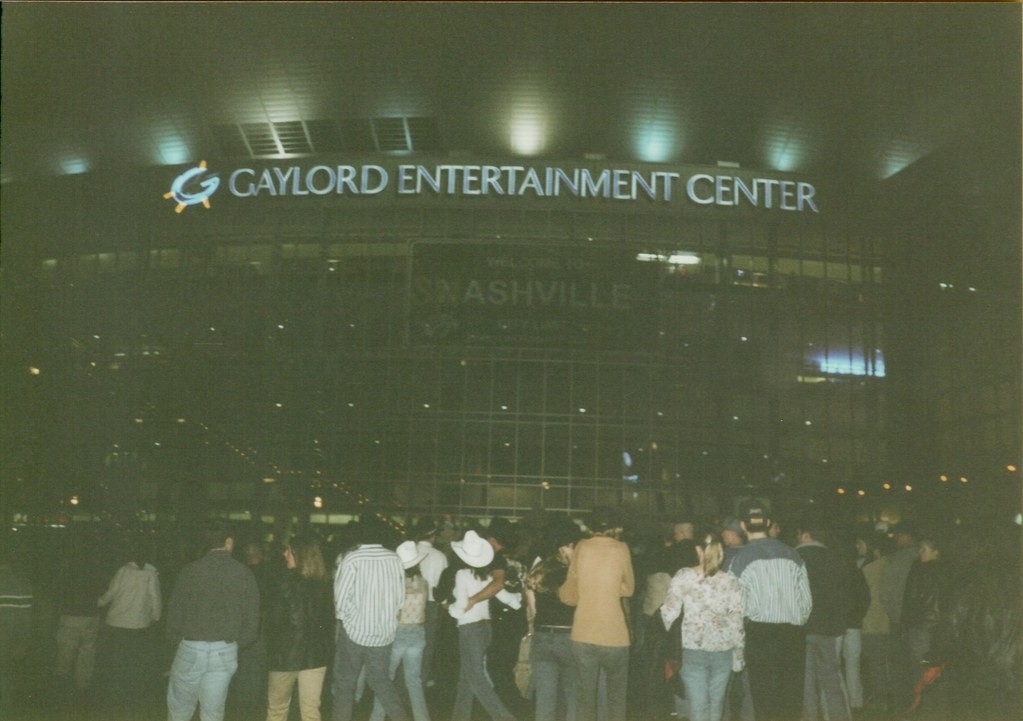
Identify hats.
[451,530,495,567]
[739,499,770,532]
[396,541,429,570]
[412,517,444,538]
[888,520,912,536]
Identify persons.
[0,500,1023,721]
